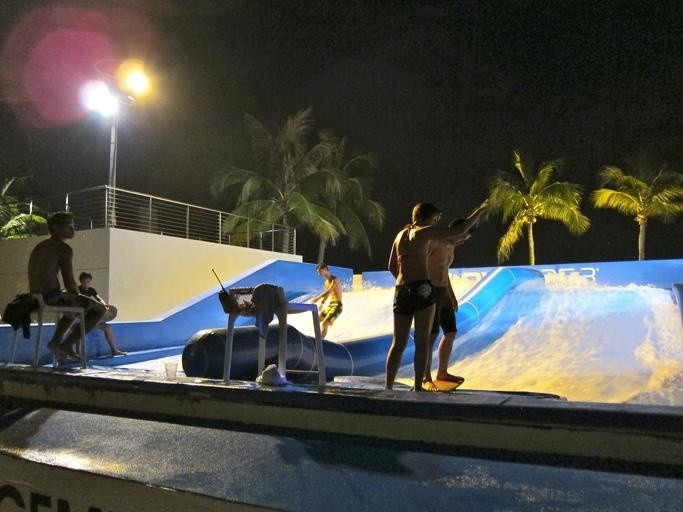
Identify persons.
[28,212,107,361]
[385,201,491,394]
[418,218,481,392]
[71,271,128,360]
[306,261,345,342]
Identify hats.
[255,365,286,386]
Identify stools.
[10,293,88,369]
[223,286,329,388]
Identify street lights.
[80,56,153,227]
